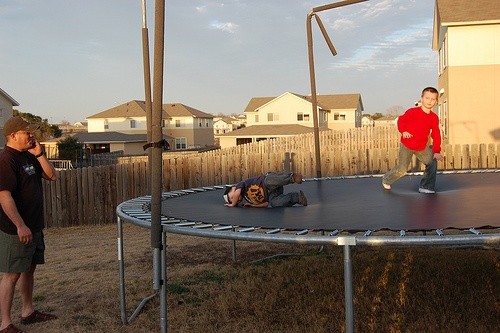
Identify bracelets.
[35,150,43,159]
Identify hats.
[4,117,30,137]
[223,187,238,207]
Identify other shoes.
[299,190,307,206]
[0,324,27,333]
[293,173,302,184]
[418,187,435,194]
[21,310,58,325]
[383,182,391,190]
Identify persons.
[223,170,308,208]
[382,87,445,193]
[0,116,59,333]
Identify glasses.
[15,131,30,135]
